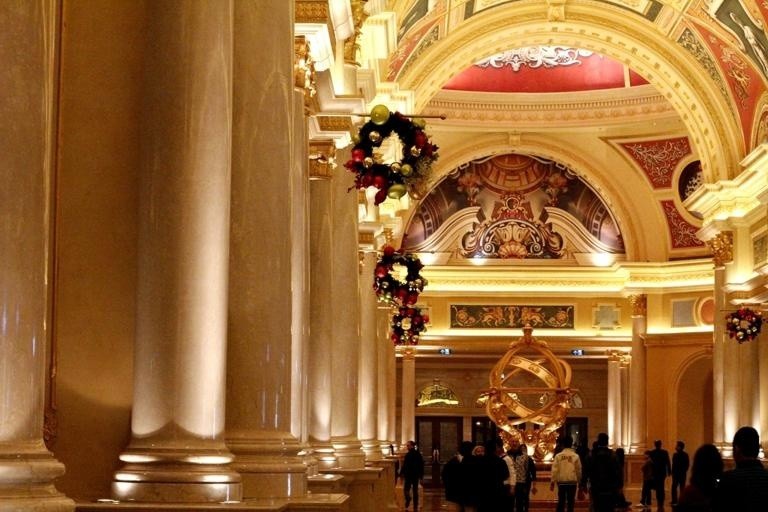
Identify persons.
[441,427,537,511]
[640,427,768,511]
[398,441,424,512]
[550,430,632,511]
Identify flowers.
[724,307,762,344]
[389,306,430,346]
[342,106,439,206]
[372,246,428,305]
